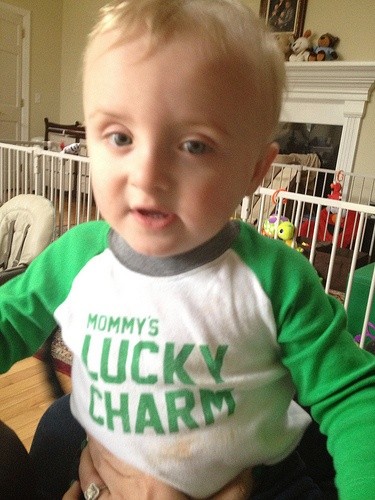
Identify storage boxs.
[313,244,375,337]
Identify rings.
[83,482,108,500]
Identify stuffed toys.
[261,214,289,240]
[277,219,303,253]
[277,29,341,63]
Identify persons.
[268,0,296,33]
[62,430,253,500]
[0,0,375,500]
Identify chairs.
[0,194,56,285]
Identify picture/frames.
[259,0,307,40]
